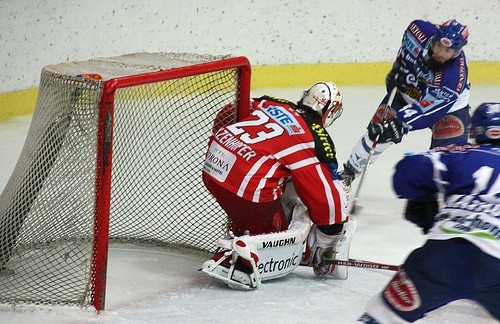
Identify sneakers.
[339,163,355,188]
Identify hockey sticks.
[348,71,405,215]
[299,251,402,272]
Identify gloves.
[404,194,439,235]
[368,117,403,144]
[385,58,407,96]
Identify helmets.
[300,81,343,128]
[469,102,500,140]
[434,19,469,49]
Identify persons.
[339,18,471,187]
[353,101,500,324]
[197,80,350,291]
